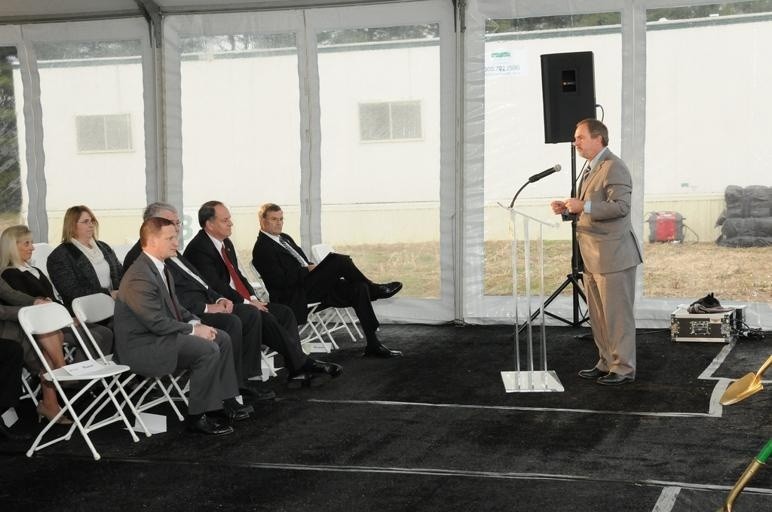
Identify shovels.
[719,354,772,406]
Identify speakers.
[539,51,598,144]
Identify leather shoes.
[598,372,635,386]
[579,367,609,378]
[210,399,254,420]
[183,412,233,437]
[307,360,343,389]
[1,425,32,442]
[239,379,276,401]
[365,344,404,359]
[369,282,402,301]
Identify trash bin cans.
[648,210,683,243]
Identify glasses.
[78,219,97,225]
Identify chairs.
[312,242,382,338]
[260,347,286,378]
[27,243,98,399]
[72,293,185,438]
[317,307,364,349]
[20,368,47,420]
[248,259,339,355]
[17,302,141,460]
[166,369,192,407]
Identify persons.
[124,202,276,414]
[182,200,344,388]
[110,216,251,437]
[550,117,644,386]
[0,225,114,400]
[46,206,123,342]
[0,339,33,455]
[252,204,402,360]
[0,278,74,425]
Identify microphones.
[529,165,561,182]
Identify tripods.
[510,142,591,339]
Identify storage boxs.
[677,305,748,336]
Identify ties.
[221,244,250,301]
[281,238,305,267]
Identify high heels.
[36,400,74,424]
